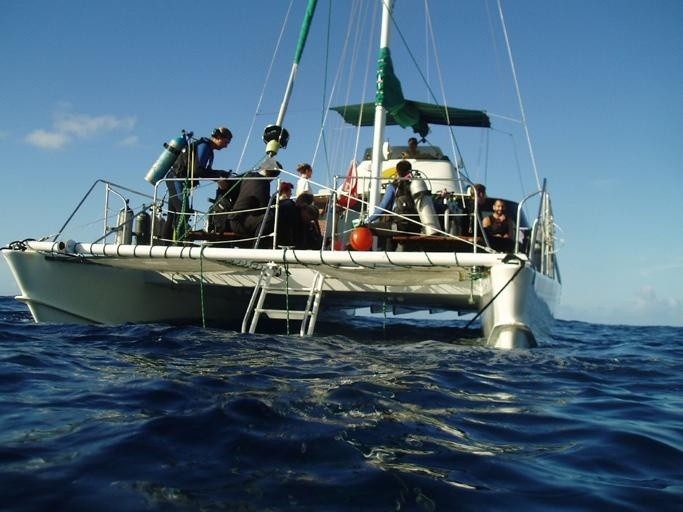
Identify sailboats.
[1,0,564,350]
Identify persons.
[156,126,234,246]
[209,159,329,251]
[400,136,429,159]
[360,159,512,251]
